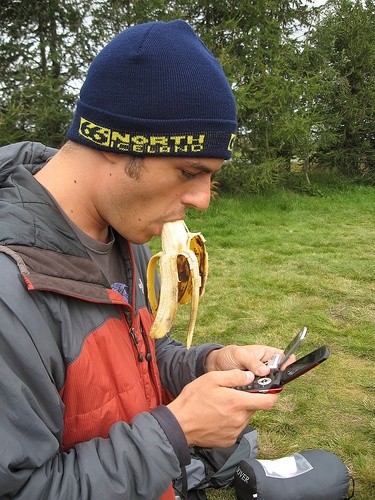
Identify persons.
[0,19,297,499]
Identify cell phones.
[228,345,329,393]
[244,327,307,373]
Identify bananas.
[146,219,210,350]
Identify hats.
[64,19,238,159]
